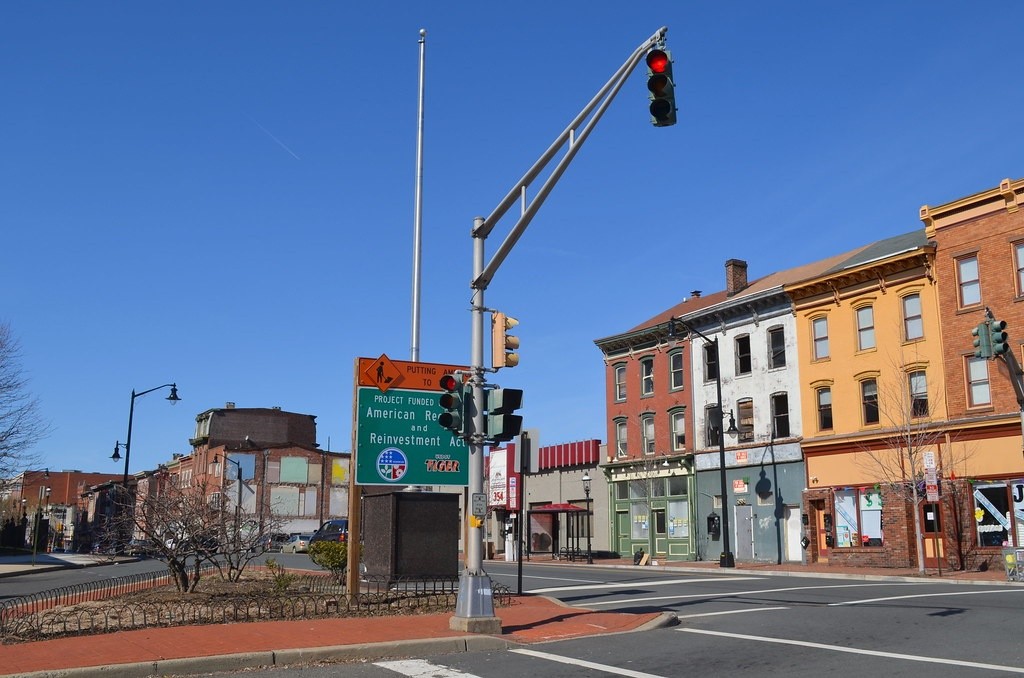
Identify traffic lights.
[643,49,677,126]
[972,323,992,359]
[989,319,1009,355]
[436,373,465,434]
[490,312,521,370]
[485,388,524,442]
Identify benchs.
[559,553,588,561]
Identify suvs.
[309,518,349,554]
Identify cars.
[254,532,290,552]
[279,533,311,554]
[53,532,220,558]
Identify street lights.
[668,315,742,569]
[108,379,182,555]
[210,452,240,554]
[43,485,53,516]
[16,467,52,526]
[580,472,594,565]
